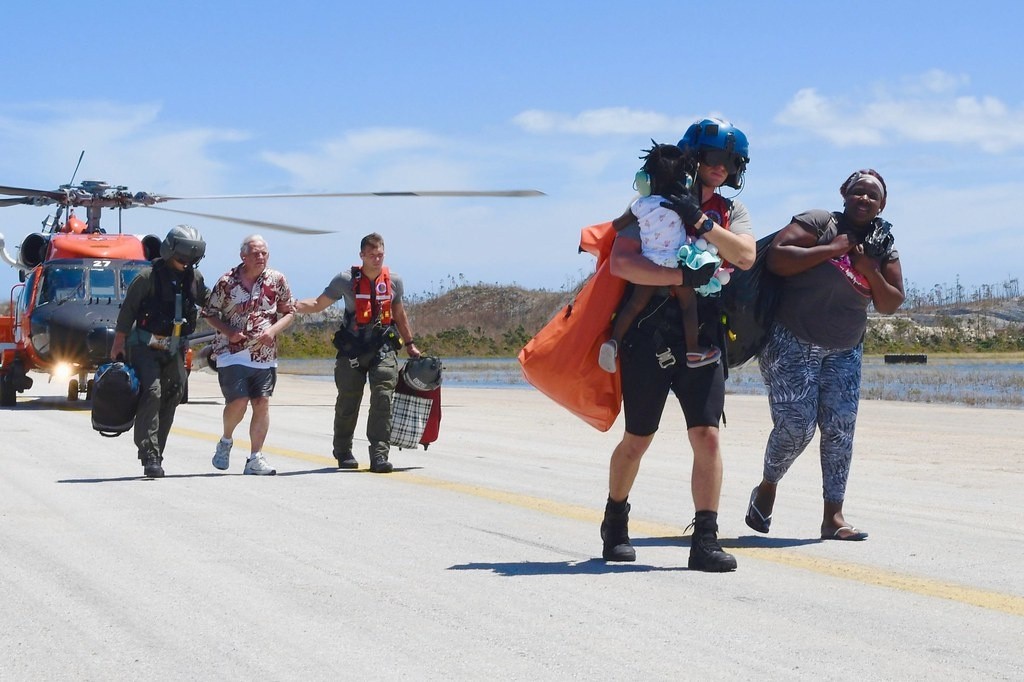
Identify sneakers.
[244,453,276,475]
[688,533,737,571]
[212,440,233,470]
[601,505,636,561]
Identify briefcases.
[396,368,440,452]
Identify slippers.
[746,487,771,533]
[820,525,868,541]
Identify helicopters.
[0,150,549,406]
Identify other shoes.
[333,450,358,470]
[144,458,165,477]
[370,455,393,474]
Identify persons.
[277,233,423,473]
[599,118,757,572]
[111,226,217,477]
[198,235,296,476]
[745,169,905,540]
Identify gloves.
[659,190,702,225]
[682,264,714,287]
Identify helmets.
[161,226,206,269]
[677,118,749,191]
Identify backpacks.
[518,221,630,431]
[724,212,893,368]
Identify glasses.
[363,253,384,262]
[699,149,740,175]
[245,250,269,258]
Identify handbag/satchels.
[389,393,433,451]
[398,349,443,391]
[91,362,140,437]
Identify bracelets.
[293,299,298,310]
[865,267,878,278]
[405,340,415,346]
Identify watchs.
[697,218,714,235]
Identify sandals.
[598,340,618,372]
[686,346,722,368]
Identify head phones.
[633,166,693,197]
